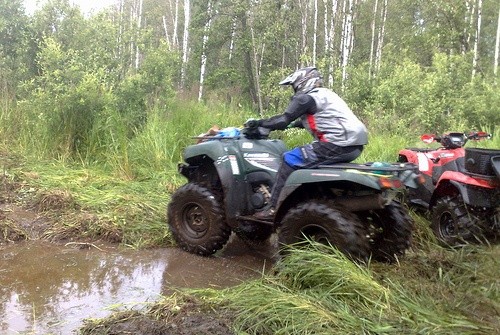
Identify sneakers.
[253,209,275,219]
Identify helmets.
[278,67,322,101]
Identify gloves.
[244,118,262,128]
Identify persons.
[243,67,368,220]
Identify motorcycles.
[168,120,413,266]
[382,131,500,249]
